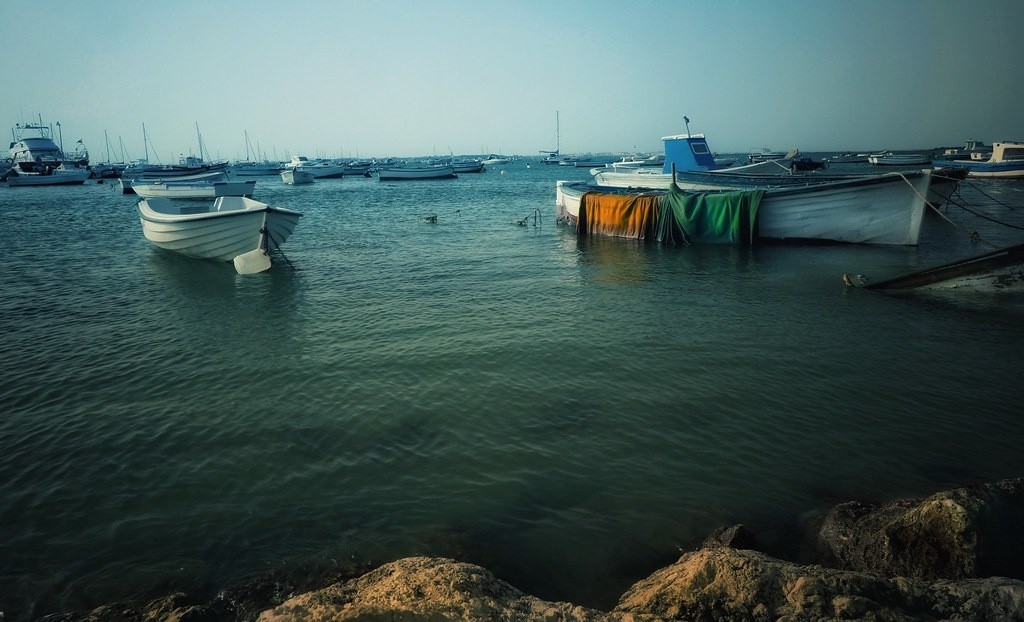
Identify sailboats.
[90,120,285,178]
[543,111,571,165]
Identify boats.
[130,179,257,198]
[118,171,226,195]
[134,196,304,275]
[1,102,91,185]
[280,154,509,185]
[557,115,1023,248]
